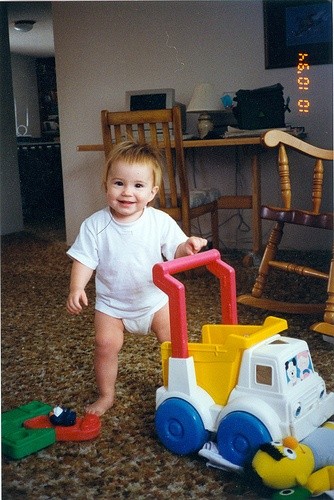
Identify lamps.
[13,22,33,33]
[186,83,227,139]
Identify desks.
[77,128,306,269]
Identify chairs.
[101,106,219,250]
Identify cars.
[150,247,334,500]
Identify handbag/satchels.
[232,82,291,130]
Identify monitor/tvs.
[124,88,187,134]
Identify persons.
[67,131,208,416]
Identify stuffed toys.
[252,414,334,500]
